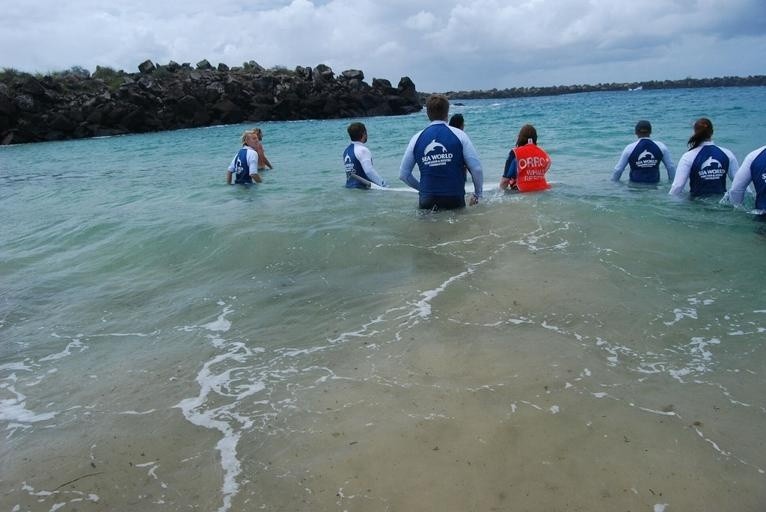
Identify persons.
[399,94,483,211]
[611,120,676,183]
[342,122,386,190]
[499,124,553,193]
[718,144,766,216]
[227,130,263,184]
[449,112,465,131]
[250,127,274,170]
[668,117,740,199]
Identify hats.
[636,121,651,133]
